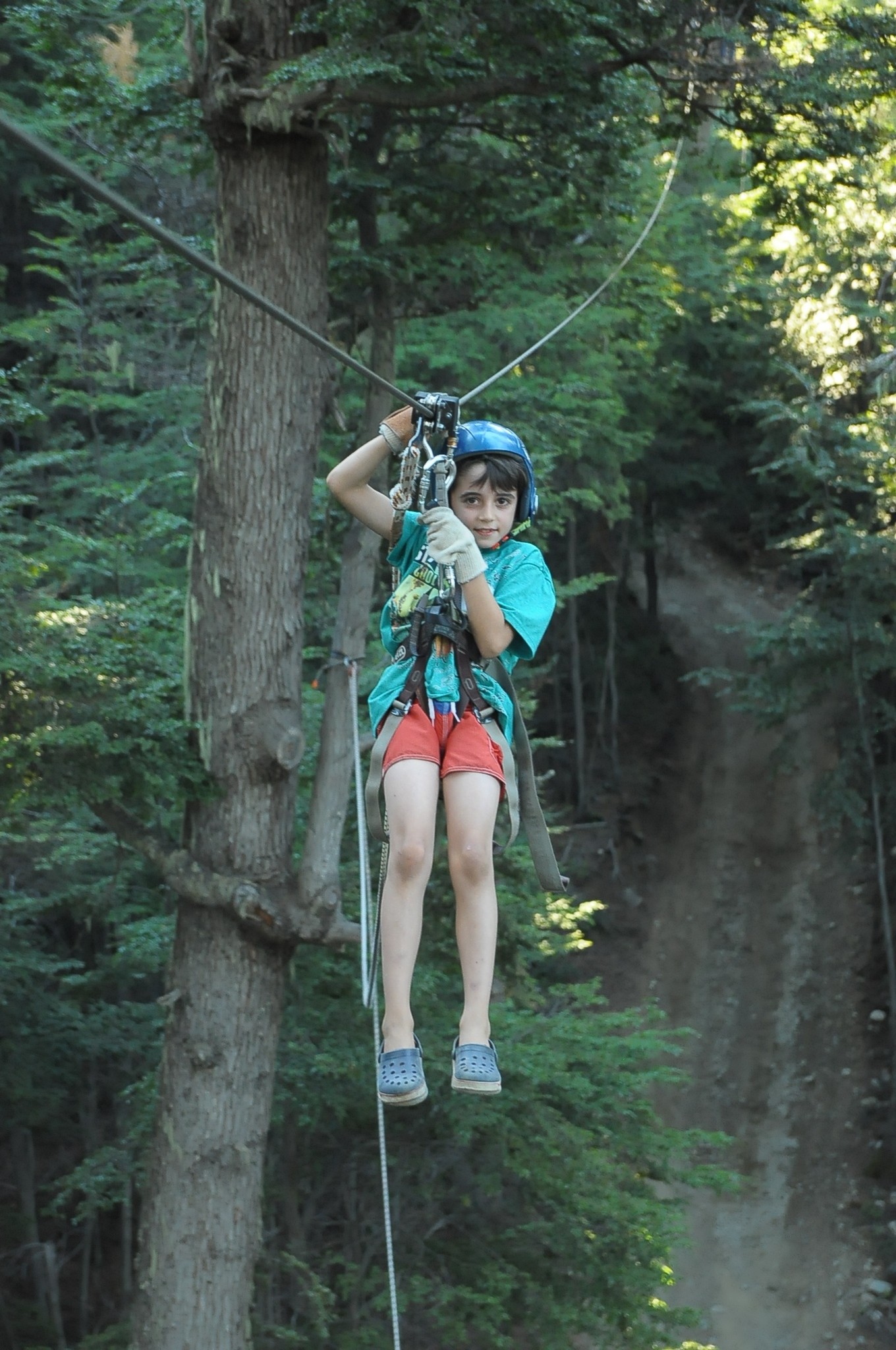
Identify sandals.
[376,1032,428,1106]
[451,1036,502,1095]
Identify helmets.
[426,420,539,524]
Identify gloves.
[418,506,489,585]
[379,393,448,454]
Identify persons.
[324,392,558,1108]
